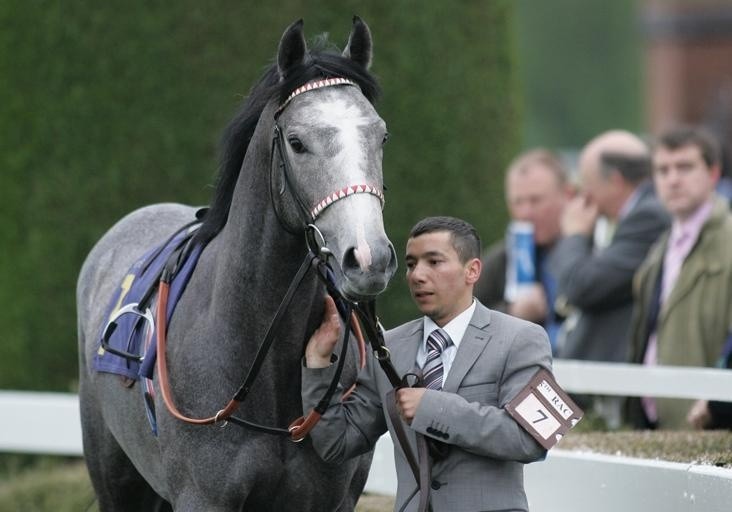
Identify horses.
[76,12,400,512]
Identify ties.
[420,329,453,460]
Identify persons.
[685,350,732,432]
[472,145,580,325]
[544,129,674,430]
[617,124,731,431]
[300,215,554,512]
